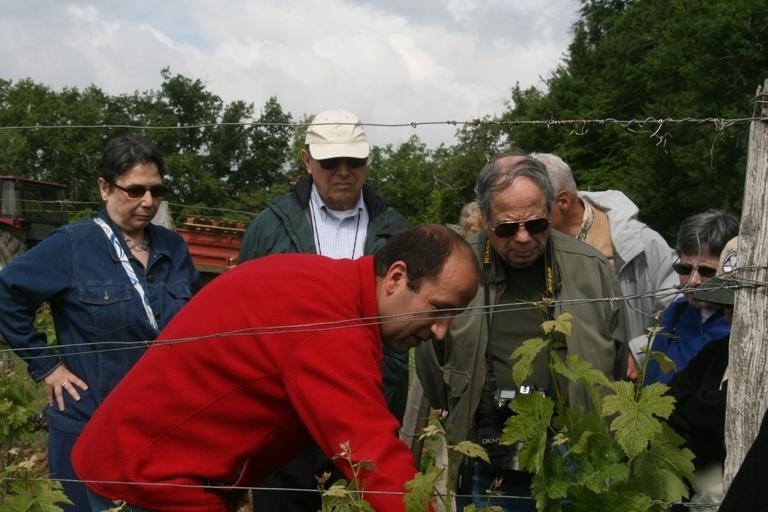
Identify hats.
[305,110,369,160]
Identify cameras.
[494,386,549,475]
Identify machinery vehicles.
[0,173,251,275]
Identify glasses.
[487,217,551,237]
[114,183,170,198]
[672,256,716,278]
[317,158,368,169]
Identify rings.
[62,380,67,388]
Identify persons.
[0,139,200,512]
